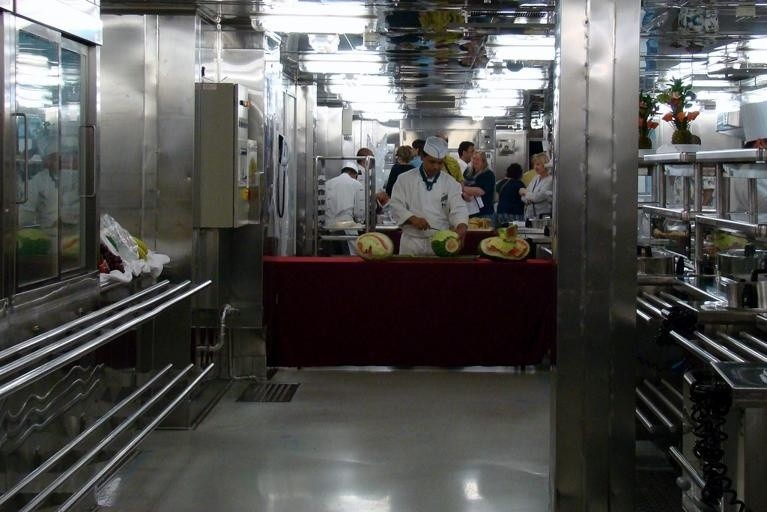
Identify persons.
[14,113,90,231]
[389,135,471,259]
[352,148,384,218]
[384,135,554,225]
[323,161,364,257]
[724,136,767,243]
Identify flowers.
[657,76,700,133]
[639,90,662,137]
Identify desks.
[322,222,501,256]
[263,255,555,371]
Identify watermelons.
[355,231,394,262]
[477,224,531,261]
[431,230,461,257]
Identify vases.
[639,136,653,149]
[673,130,692,145]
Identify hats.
[742,100,767,146]
[422,136,449,159]
[341,161,358,174]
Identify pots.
[713,243,766,314]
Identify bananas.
[132,237,148,262]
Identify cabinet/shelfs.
[2,11,97,304]
[636,147,766,318]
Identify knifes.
[427,226,440,232]
